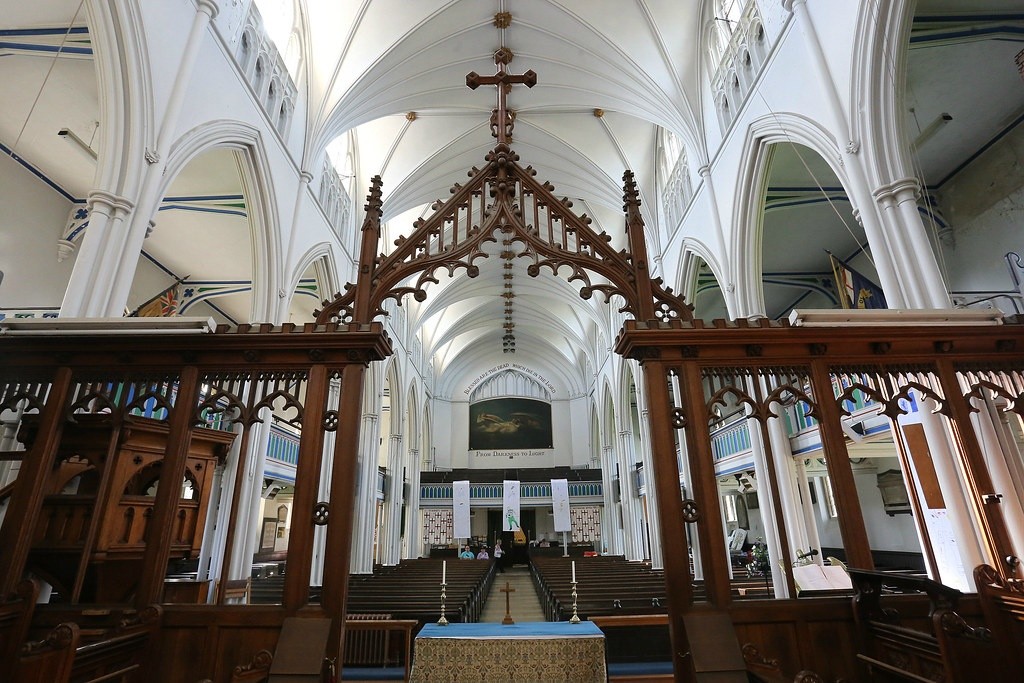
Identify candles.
[442,560,446,583]
[572,561,576,581]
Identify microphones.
[799,549,818,559]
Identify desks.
[409,622,609,683]
[164,578,212,604]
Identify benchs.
[253,557,495,665]
[0,579,163,683]
[530,552,1024,683]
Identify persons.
[506,508,520,530]
[477,547,489,559]
[492,539,506,573]
[460,545,474,560]
[540,538,549,547]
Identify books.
[792,564,853,591]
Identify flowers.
[745,537,771,579]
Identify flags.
[839,260,888,309]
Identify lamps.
[842,420,866,443]
[0,317,216,335]
[261,484,282,500]
[788,309,1005,327]
[58,122,99,165]
[740,475,758,493]
[906,107,953,154]
